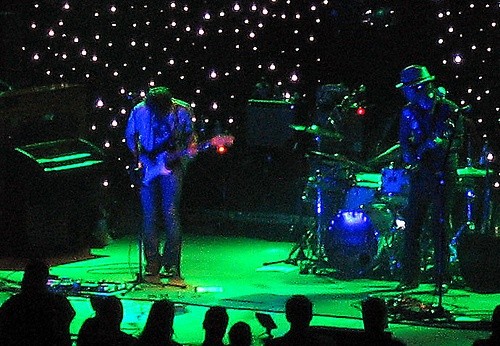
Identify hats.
[394,65,435,90]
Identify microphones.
[454,104,474,113]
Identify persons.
[0,259,500,346]
[125,87,194,288]
[396,65,463,293]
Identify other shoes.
[435,282,448,293]
[396,279,418,292]
[145,257,163,275]
[164,265,180,277]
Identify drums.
[355,173,382,181]
[301,159,357,213]
[343,180,379,208]
[323,207,394,272]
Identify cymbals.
[368,143,400,164]
[311,150,371,172]
[288,124,344,139]
[457,169,493,177]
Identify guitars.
[137,134,235,187]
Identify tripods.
[122,132,188,296]
